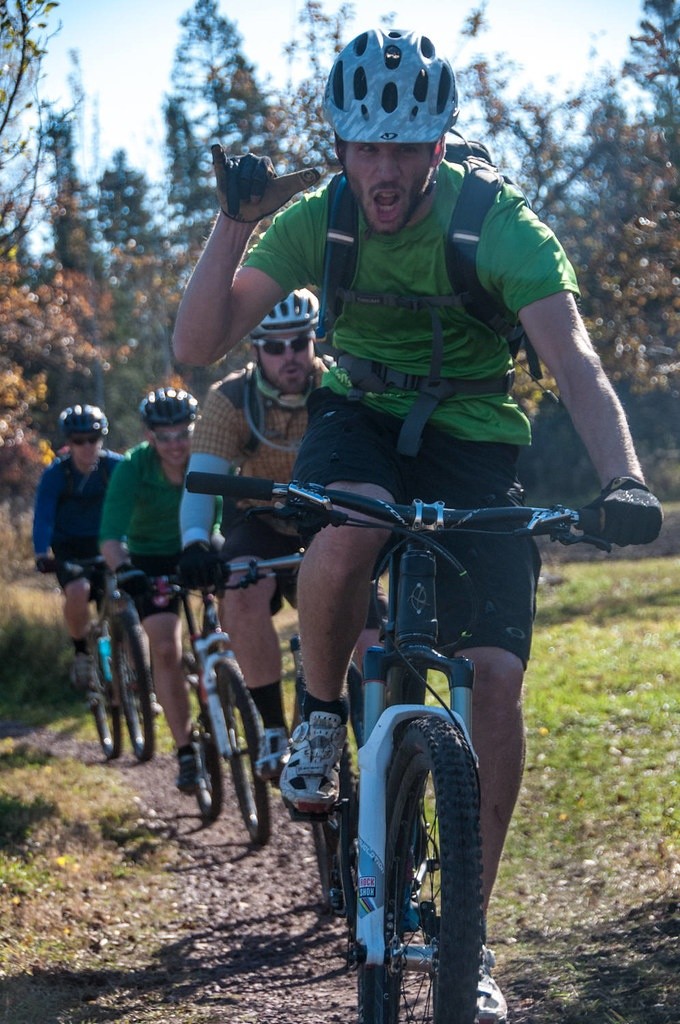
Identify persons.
[98,385,250,795]
[170,29,663,1024]
[31,405,164,719]
[179,288,391,780]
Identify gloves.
[176,541,231,592]
[116,564,151,597]
[212,144,320,221]
[583,476,663,546]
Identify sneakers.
[474,945,507,1024]
[175,745,201,795]
[69,652,106,705]
[280,711,347,813]
[256,729,289,780]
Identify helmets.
[321,29,460,142]
[59,404,109,435]
[140,387,198,426]
[250,288,320,335]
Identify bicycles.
[34,471,610,1024]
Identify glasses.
[254,332,316,354]
[149,430,193,443]
